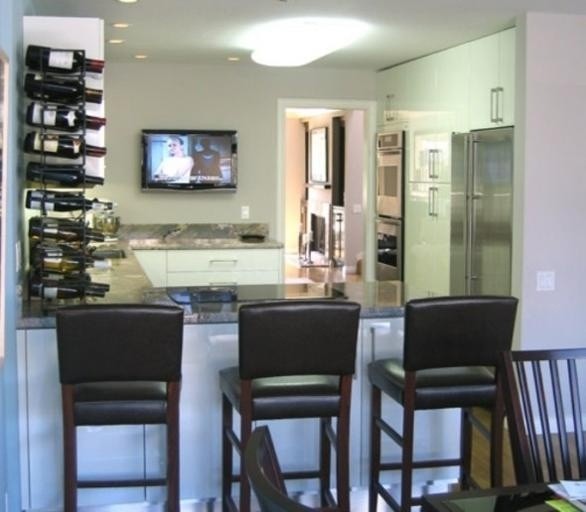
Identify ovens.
[373,148,402,281]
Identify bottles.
[22,41,126,300]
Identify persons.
[153,135,194,183]
[191,137,225,185]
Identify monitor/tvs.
[141,130,238,190]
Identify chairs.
[218,300,361,512]
[368,295,586,512]
[55,303,183,511]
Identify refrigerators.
[402,128,472,302]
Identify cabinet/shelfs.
[403,134,466,185]
[406,39,471,131]
[37,43,87,299]
[470,27,515,129]
[374,64,406,130]
[131,250,280,287]
[402,184,466,304]
